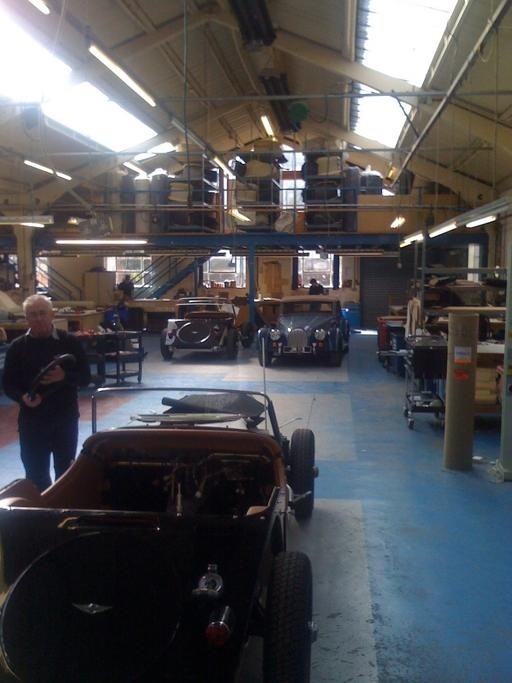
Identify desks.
[129,299,176,327]
[0,319,68,333]
[14,308,105,331]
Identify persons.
[3,294,90,491]
[122,275,134,297]
[309,279,323,311]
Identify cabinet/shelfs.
[77,329,144,387]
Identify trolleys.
[396,332,448,434]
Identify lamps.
[390,195,509,248]
[260,116,278,142]
[84,37,156,108]
[22,157,72,181]
[28,0,51,17]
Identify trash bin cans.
[340,308,351,338]
[377,315,407,376]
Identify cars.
[0,382,323,680]
[158,294,253,361]
[256,295,353,366]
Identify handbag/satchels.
[41,377,78,414]
